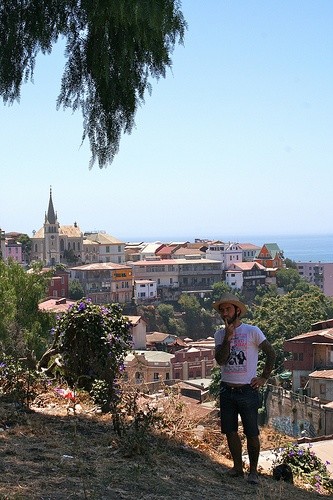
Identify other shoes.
[247,469,261,485]
[228,467,244,477]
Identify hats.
[212,293,247,320]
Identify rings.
[256,385,259,387]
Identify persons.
[213,292,276,484]
